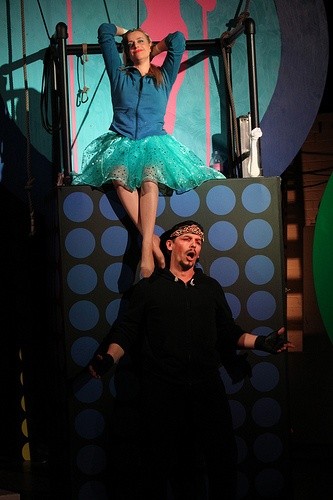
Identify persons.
[69,24,187,279]
[92,220,298,500]
